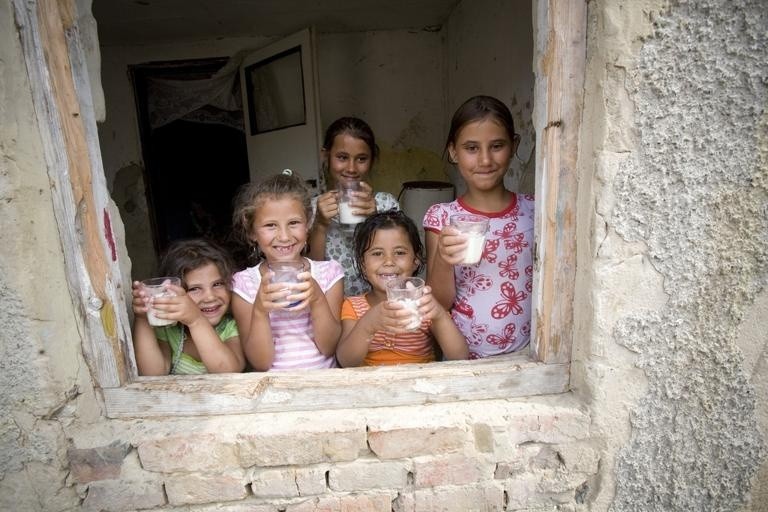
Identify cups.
[449,213,491,267]
[141,276,178,328]
[384,277,425,333]
[327,179,368,225]
[269,262,304,307]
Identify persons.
[422,94,536,363]
[128,240,246,377]
[302,117,399,295]
[229,170,345,373]
[334,208,470,369]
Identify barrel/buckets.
[401,182,457,286]
